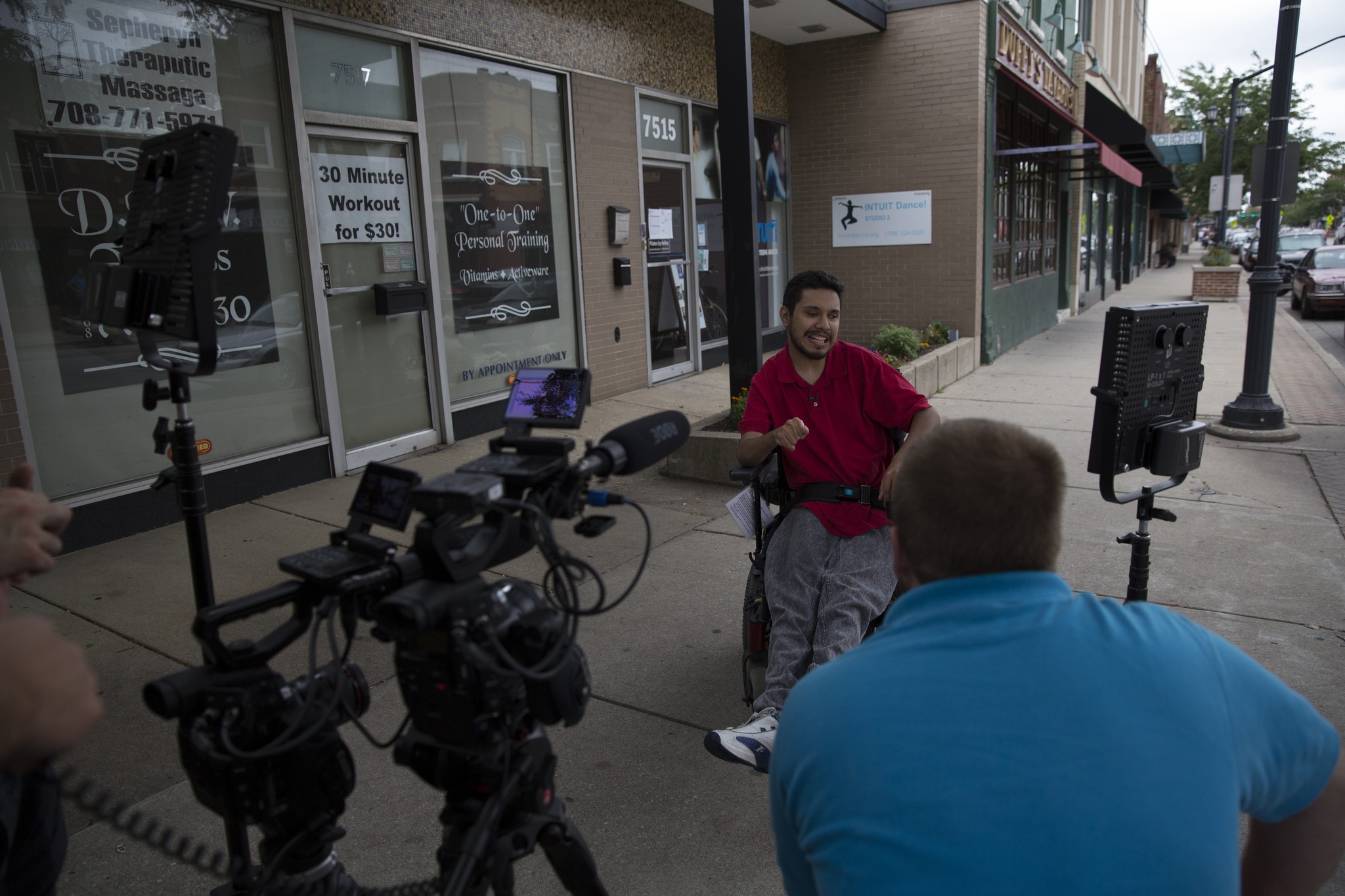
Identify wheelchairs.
[737,430,908,713]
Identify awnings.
[993,59,1143,188]
[1083,78,1188,220]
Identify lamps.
[1024,0,1102,79]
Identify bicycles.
[142,363,688,896]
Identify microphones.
[568,410,690,480]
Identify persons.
[0,396,107,895]
[764,415,1345,896]
[703,269,940,775]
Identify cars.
[1290,245,1345,318]
[1199,225,1329,297]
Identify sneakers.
[704,708,780,775]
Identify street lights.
[1204,103,1248,198]
